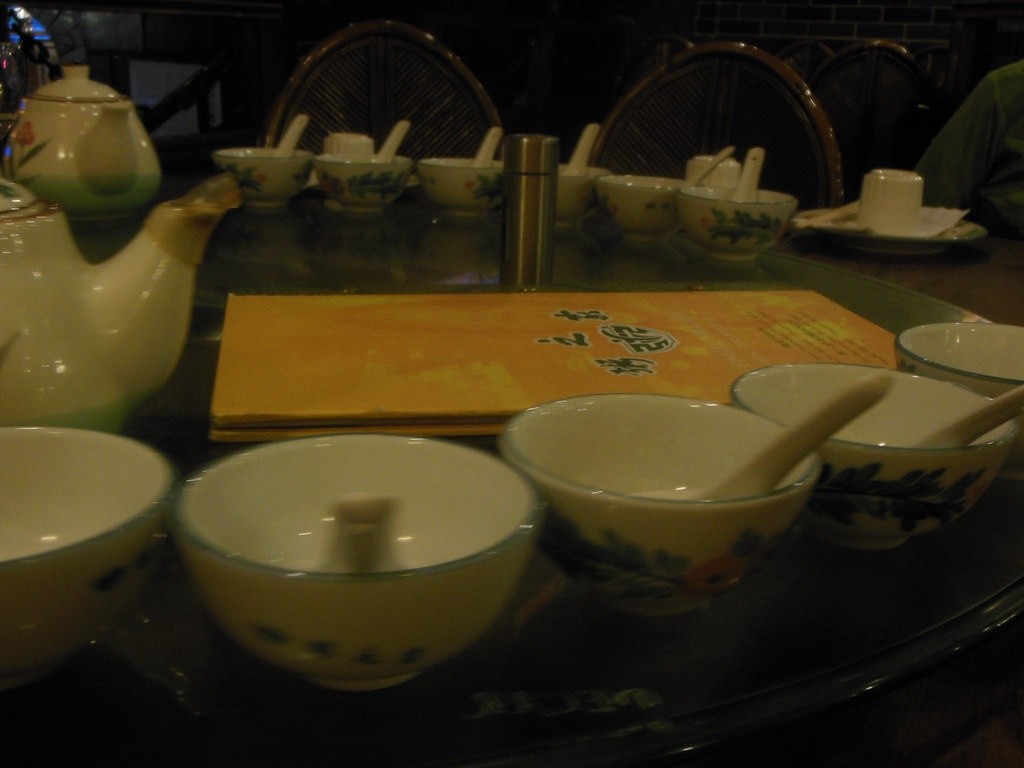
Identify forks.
[794,201,858,229]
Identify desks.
[1,157,1024,768]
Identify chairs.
[263,20,510,177]
[591,42,935,209]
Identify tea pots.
[4,65,161,219]
[0,177,243,429]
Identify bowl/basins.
[730,360,1020,547]
[314,154,412,217]
[0,423,174,694]
[417,157,504,219]
[894,321,1024,482]
[555,164,611,229]
[674,185,798,260]
[594,174,684,242]
[497,393,821,620]
[177,436,543,690]
[213,148,314,207]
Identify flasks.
[498,133,560,294]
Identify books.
[205,289,900,443]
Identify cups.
[686,154,740,188]
[323,132,374,154]
[857,169,924,237]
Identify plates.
[793,208,988,256]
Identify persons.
[921,60,1024,241]
